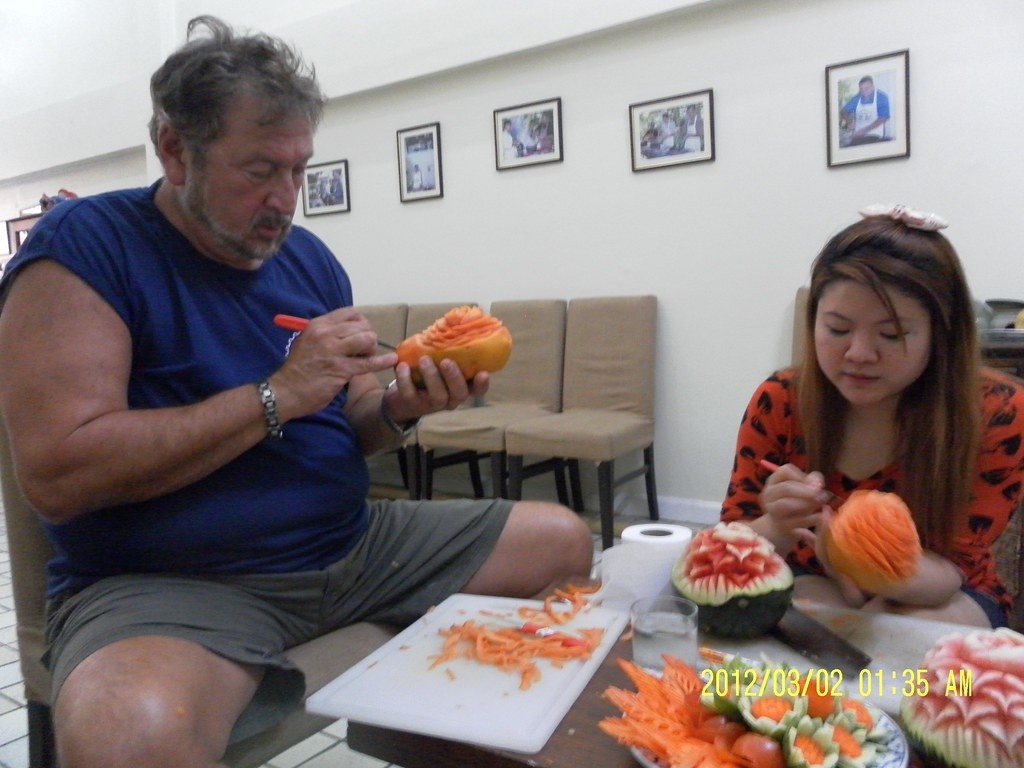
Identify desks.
[258,573,1024,768]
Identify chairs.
[0,418,403,768]
[352,295,659,550]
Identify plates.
[620,685,909,768]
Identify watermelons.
[670,521,796,639]
[900,625,1024,768]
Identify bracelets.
[382,378,422,436]
[257,382,282,439]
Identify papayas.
[393,306,512,388]
[825,490,921,594]
[596,652,872,768]
[429,578,608,690]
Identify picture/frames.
[396,122,443,202]
[629,88,715,172]
[302,159,350,216]
[825,49,910,168]
[493,96,563,170]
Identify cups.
[629,598,699,681]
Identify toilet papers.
[600,523,693,604]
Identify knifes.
[761,461,846,510]
[768,607,871,683]
[274,314,399,352]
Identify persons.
[412,165,422,190]
[326,168,343,204]
[501,120,518,157]
[0,18,596,768]
[840,76,891,140]
[641,105,704,157]
[719,206,1024,628]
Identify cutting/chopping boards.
[631,574,997,720]
[304,594,628,754]
